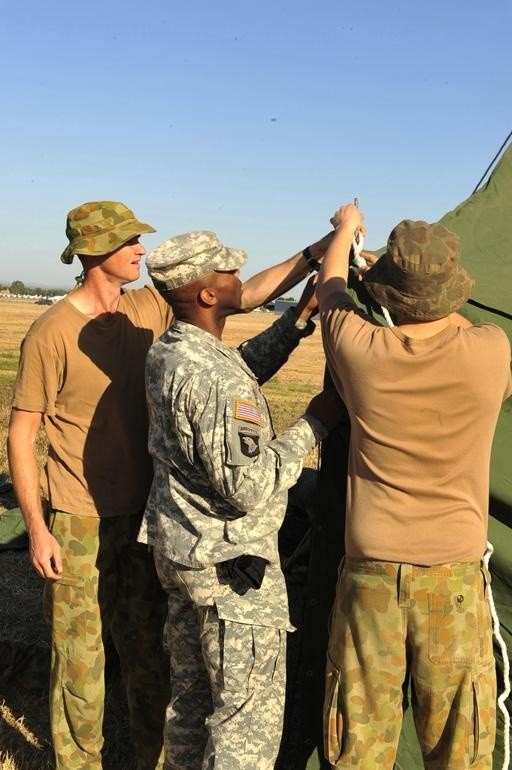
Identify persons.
[7,201,368,770]
[138,230,339,770]
[315,203,512,770]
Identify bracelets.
[301,246,320,272]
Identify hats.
[144,229,248,294]
[60,199,157,265]
[363,219,477,322]
